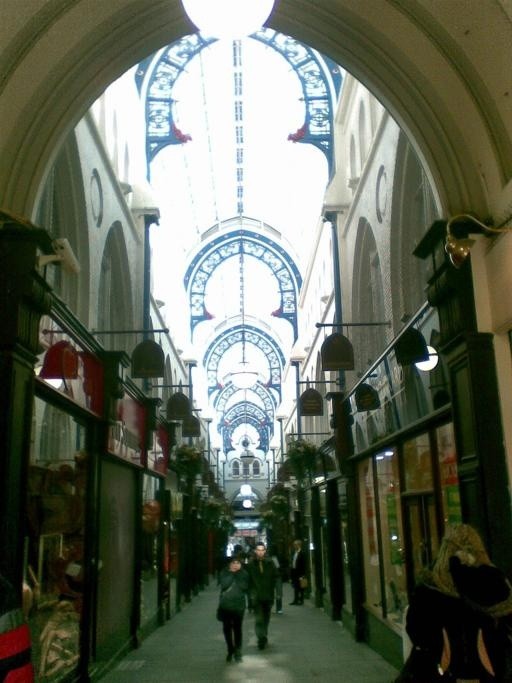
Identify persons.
[217,539,307,664]
[394,522,512,683]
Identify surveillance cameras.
[52,238,81,272]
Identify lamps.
[440,212,510,269]
